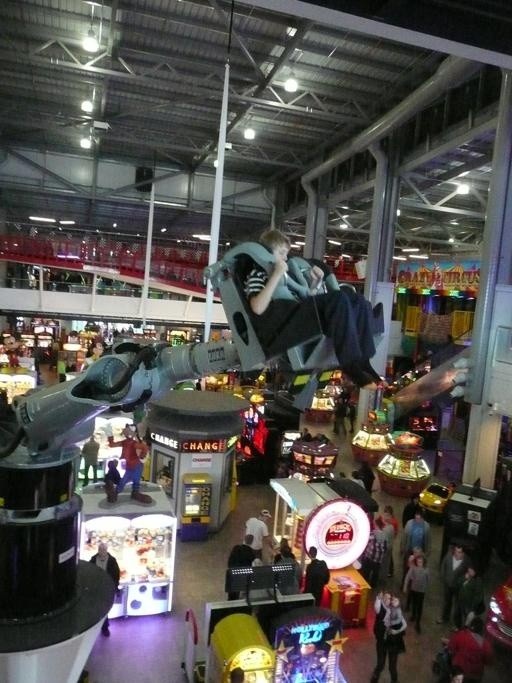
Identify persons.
[81,436,100,486]
[338,459,494,683]
[89,544,120,638]
[244,229,386,390]
[333,389,356,436]
[100,422,154,504]
[226,509,330,608]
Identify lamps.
[243,118,255,139]
[81,5,99,54]
[284,66,298,93]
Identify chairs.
[201,241,387,373]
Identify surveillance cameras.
[487,401,497,411]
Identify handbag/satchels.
[436,653,452,675]
[474,601,485,615]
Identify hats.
[260,509,272,518]
